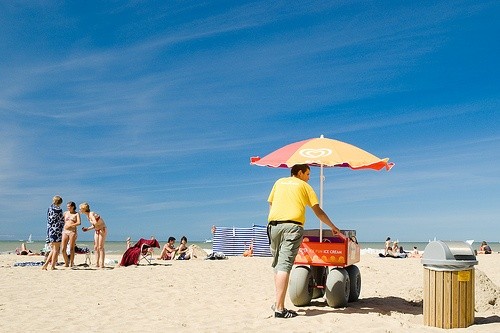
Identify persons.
[266,165,340,318]
[42,195,65,270]
[15,243,36,256]
[79,202,107,268]
[62,201,81,267]
[126,237,132,248]
[477,241,491,254]
[147,235,155,257]
[385,237,419,258]
[161,236,193,260]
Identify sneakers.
[275,308,298,318]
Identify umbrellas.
[250,135,395,242]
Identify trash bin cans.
[419,239,479,329]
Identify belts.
[269,220,303,226]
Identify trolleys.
[289,229,361,307]
